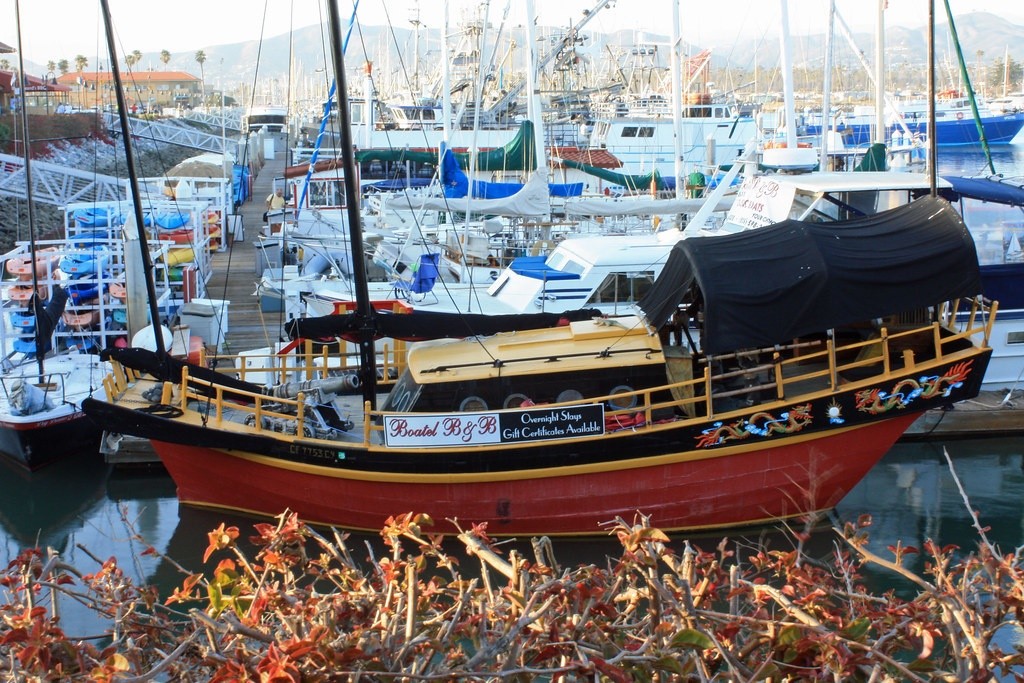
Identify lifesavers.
[298,250,358,286]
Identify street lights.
[44,71,58,115]
[82,80,96,110]
[76,75,88,112]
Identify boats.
[0,449,177,573]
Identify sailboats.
[82,1,998,548]
[0,4,1024,468]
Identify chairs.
[389,252,440,307]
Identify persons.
[56,101,72,114]
[131,103,138,114]
[265,187,295,235]
